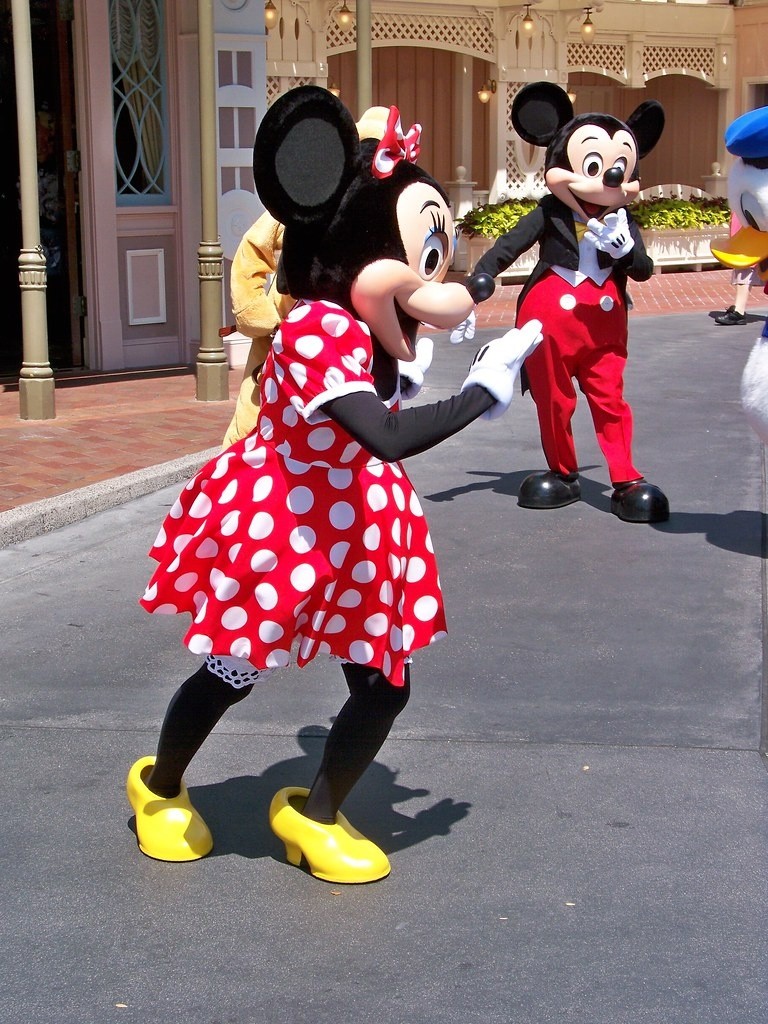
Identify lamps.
[338,0,353,33]
[581,7,595,45]
[520,4,536,37]
[567,88,577,103]
[478,78,496,103]
[264,0,280,29]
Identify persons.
[35,102,73,367]
[715,211,764,325]
[223,105,403,450]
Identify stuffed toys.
[126,86,544,886]
[710,105,768,445]
[451,82,670,523]
